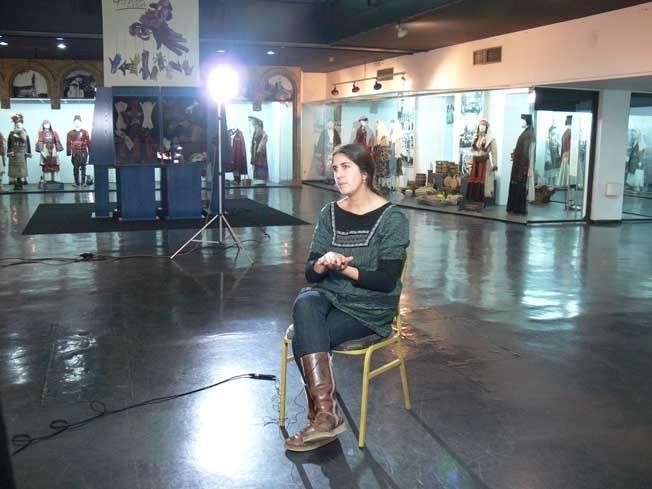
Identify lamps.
[328,59,411,98]
[167,66,258,267]
[395,20,408,39]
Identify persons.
[556,115,581,212]
[36,120,64,182]
[7,114,32,190]
[283,143,409,453]
[466,120,498,206]
[226,128,248,182]
[248,114,268,181]
[312,119,341,185]
[505,113,536,216]
[625,129,646,194]
[66,115,92,189]
[353,115,375,163]
[544,126,560,187]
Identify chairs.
[279,244,413,448]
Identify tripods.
[170,103,254,265]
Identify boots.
[283,383,338,452]
[299,351,347,445]
